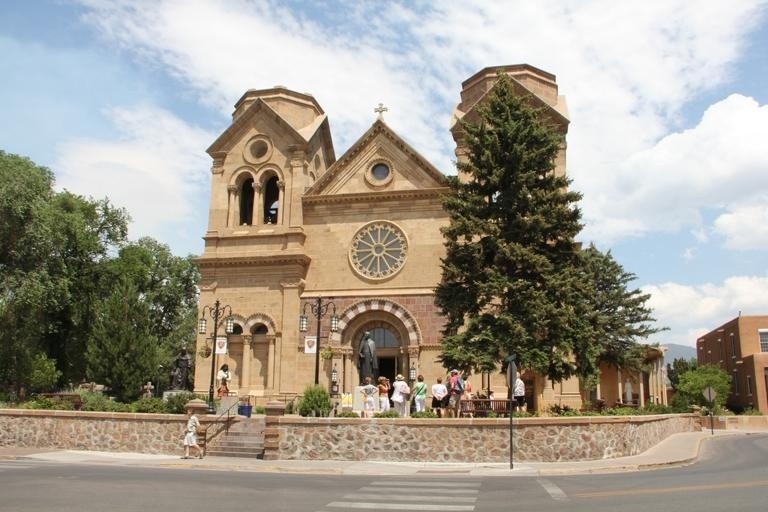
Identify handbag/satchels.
[412,396,415,405]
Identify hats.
[395,374,405,380]
[451,369,459,373]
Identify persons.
[411,374,428,414]
[624,377,634,404]
[179,407,205,460]
[431,377,447,418]
[357,330,379,386]
[390,374,411,418]
[513,370,527,414]
[459,375,475,418]
[445,375,453,417]
[168,344,192,391]
[487,390,495,410]
[378,376,391,413]
[216,363,232,396]
[447,368,461,418]
[360,376,375,418]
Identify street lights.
[299,296,339,386]
[198,298,234,414]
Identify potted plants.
[201,344,211,358]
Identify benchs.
[459,399,516,417]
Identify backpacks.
[454,376,464,394]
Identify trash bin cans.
[220,391,238,416]
[328,394,342,417]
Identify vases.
[238,405,252,417]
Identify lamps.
[408,364,415,380]
[332,368,338,384]
